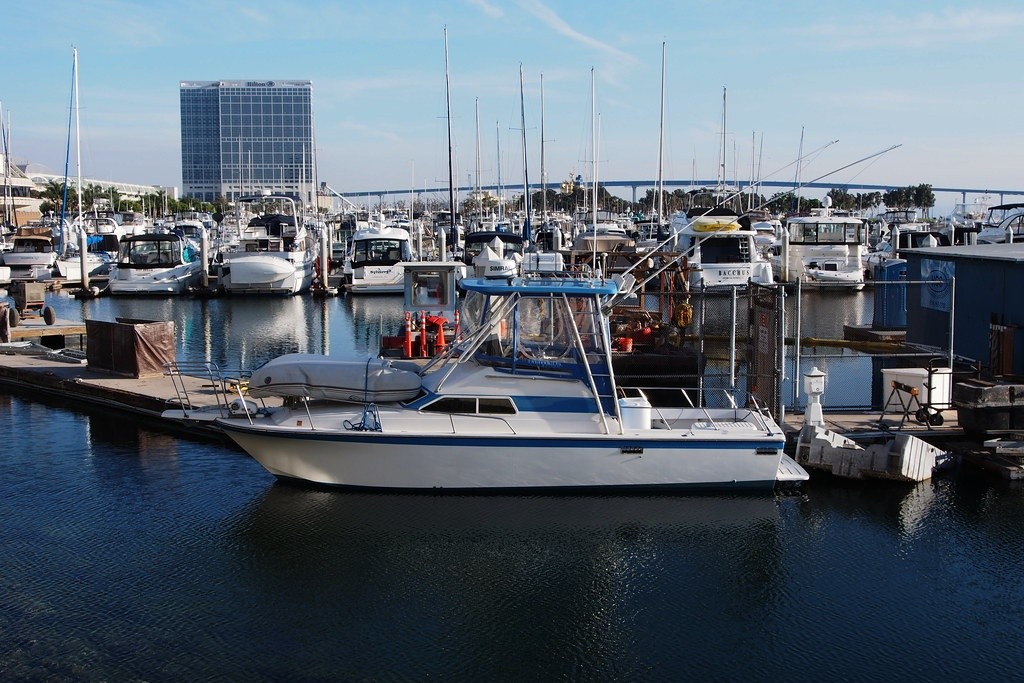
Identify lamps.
[803,367,826,404]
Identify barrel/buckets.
[620,405,652,430]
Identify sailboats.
[423,23,1023,296]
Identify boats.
[1,190,416,298]
[161,139,904,495]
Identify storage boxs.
[524,252,563,271]
[619,397,652,428]
[881,367,952,411]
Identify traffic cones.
[405,312,412,358]
[434,324,449,347]
[454,310,461,339]
[420,310,426,357]
